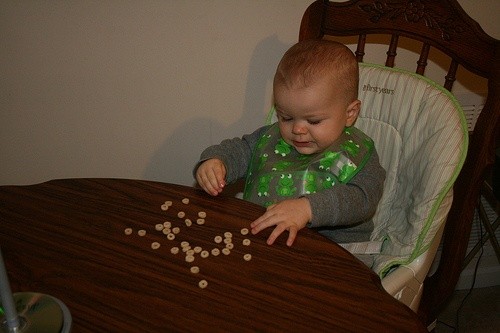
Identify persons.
[193,40,386,267]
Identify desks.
[0,178,429,333]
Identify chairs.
[298,0,500,326]
